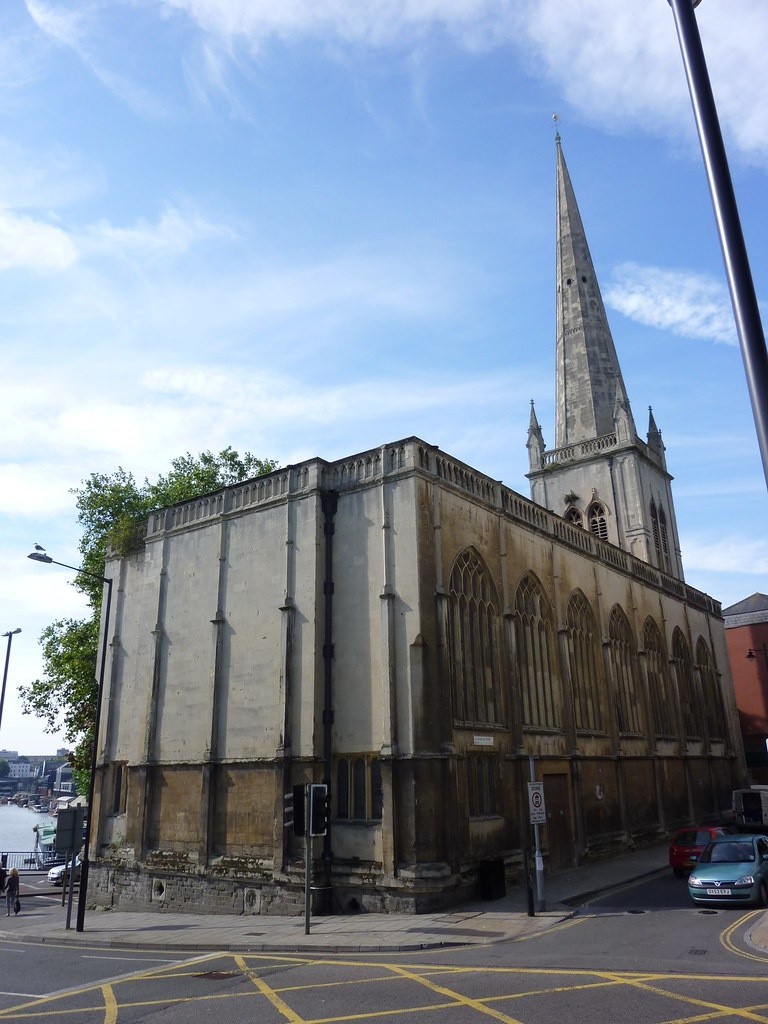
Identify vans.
[731,787,768,832]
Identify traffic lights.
[282,783,305,837]
[310,784,332,837]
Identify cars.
[669,826,737,880]
[45,854,83,887]
[686,835,768,906]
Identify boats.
[32,819,87,869]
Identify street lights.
[26,552,114,931]
[0,627,22,731]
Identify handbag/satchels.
[14,899,20,913]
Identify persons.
[4,868,20,916]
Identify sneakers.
[5,913,10,916]
[14,912,18,916]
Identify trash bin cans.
[480,858,506,901]
